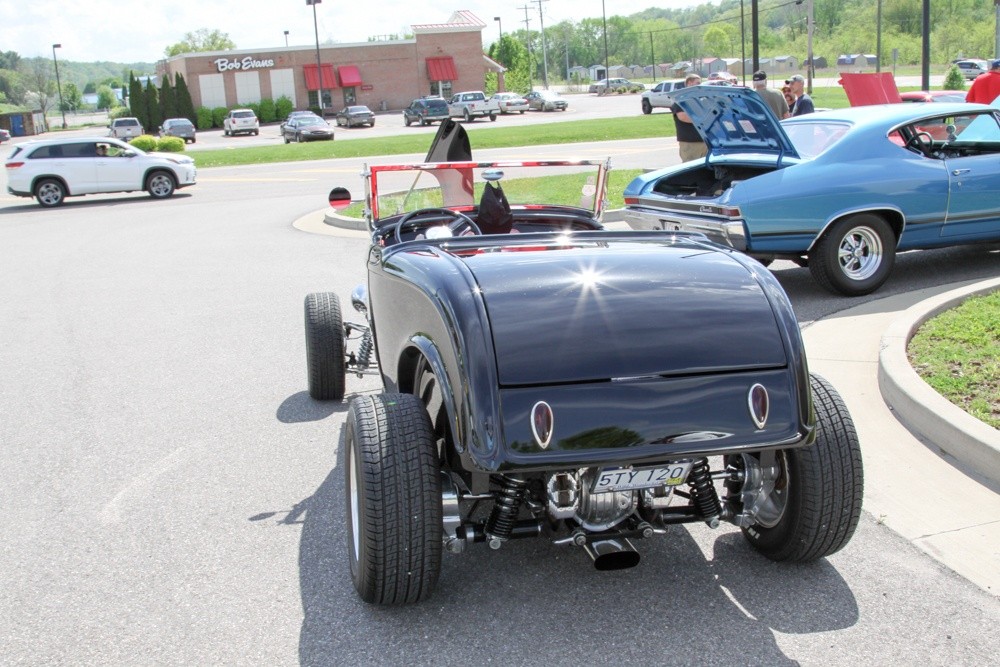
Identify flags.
[425,122,475,209]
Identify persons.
[97,145,109,157]
[965,59,1000,119]
[781,83,795,114]
[751,69,790,121]
[790,76,815,115]
[671,74,708,162]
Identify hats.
[477,179,513,234]
[785,75,804,85]
[753,71,767,81]
[992,59,1000,66]
[782,86,791,91]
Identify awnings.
[337,65,361,87]
[425,55,459,82]
[301,63,338,90]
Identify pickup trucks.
[447,90,502,123]
[641,79,690,114]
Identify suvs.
[3,133,196,208]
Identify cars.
[305,154,865,603]
[952,59,988,79]
[590,77,645,94]
[158,117,197,143]
[223,108,260,137]
[522,89,570,112]
[0,127,9,144]
[622,72,999,297]
[111,117,145,141]
[336,105,375,128]
[489,91,530,114]
[402,97,453,126]
[281,112,336,142]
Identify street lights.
[52,43,69,128]
[306,0,325,118]
[494,16,504,68]
[284,31,289,47]
